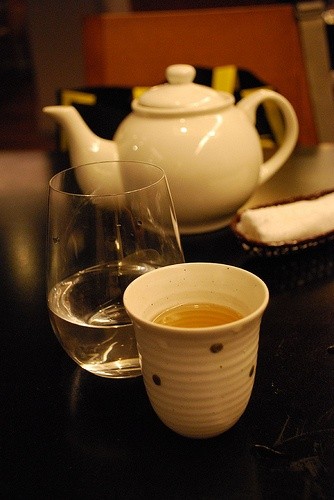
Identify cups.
[123,262,270,438]
[45,161,186,378]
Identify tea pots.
[42,62,299,235]
